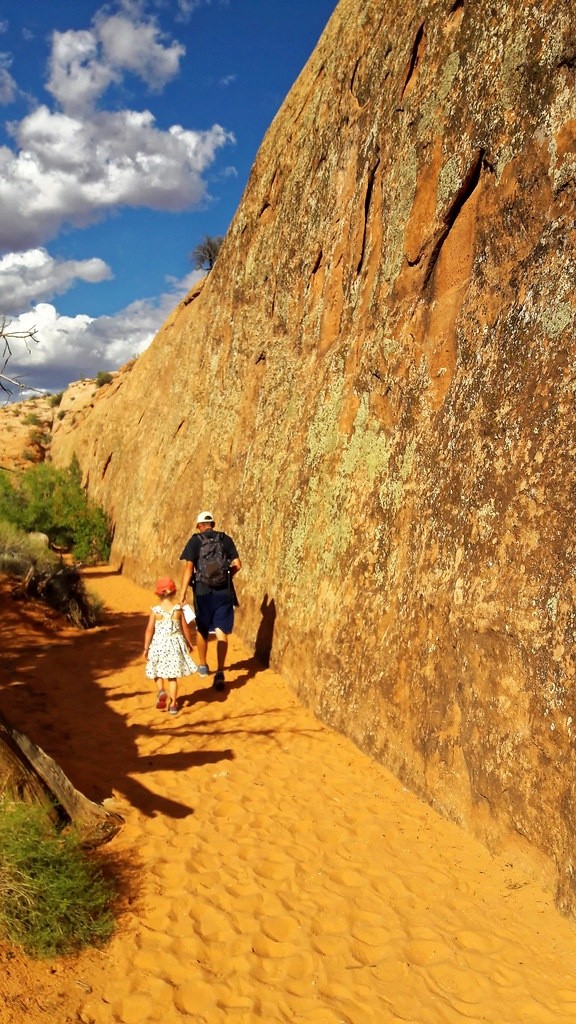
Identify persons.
[142,576,198,714]
[179,511,242,691]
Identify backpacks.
[188,531,232,587]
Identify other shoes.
[156,691,168,709]
[197,666,209,677]
[167,704,178,714]
[213,673,225,690]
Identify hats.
[196,511,215,528]
[154,580,177,596]
